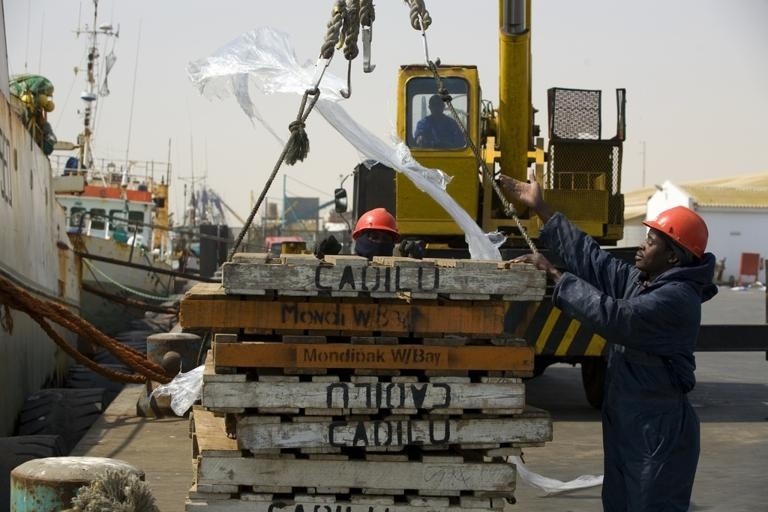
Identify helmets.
[351,206,402,240]
[641,205,710,261]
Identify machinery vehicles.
[335,0,641,410]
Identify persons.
[412,92,466,149]
[496,168,718,512]
[311,206,427,260]
